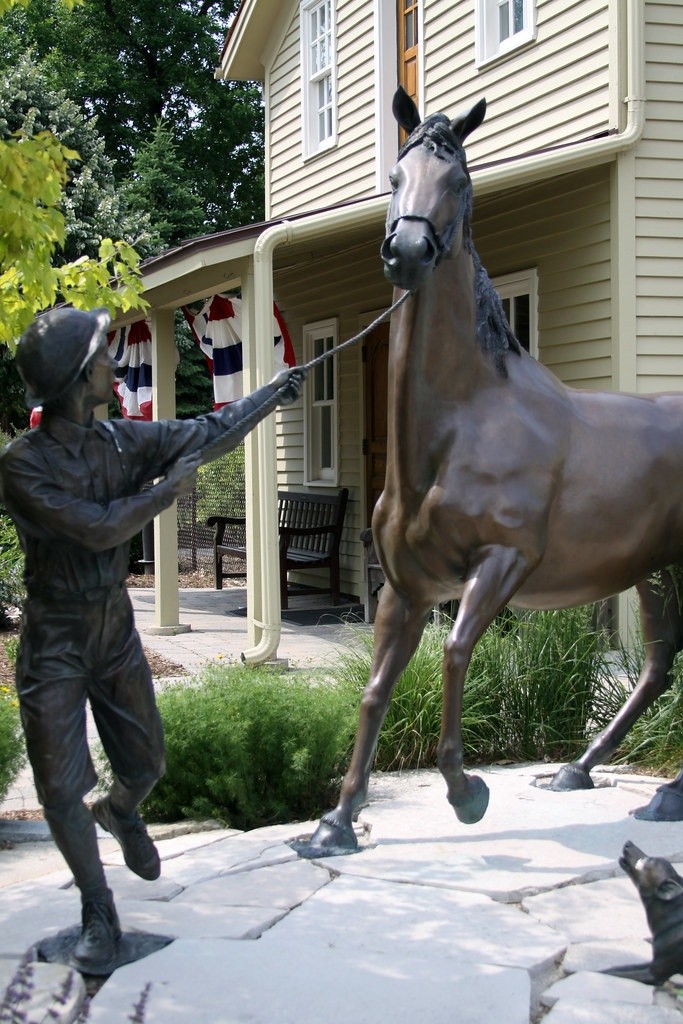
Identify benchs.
[202,487,348,608]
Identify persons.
[0,307,307,974]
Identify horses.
[310,86,683,849]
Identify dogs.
[619,840,683,985]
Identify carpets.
[225,604,375,626]
